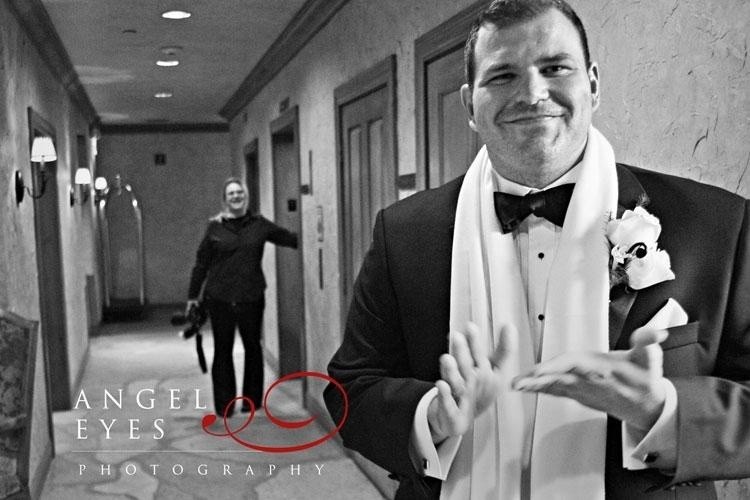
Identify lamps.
[70,168,92,207]
[94,177,107,204]
[16,136,57,203]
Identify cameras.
[170,301,208,342]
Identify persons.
[185,177,299,425]
[322,0,750,500]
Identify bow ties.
[492,183,574,233]
